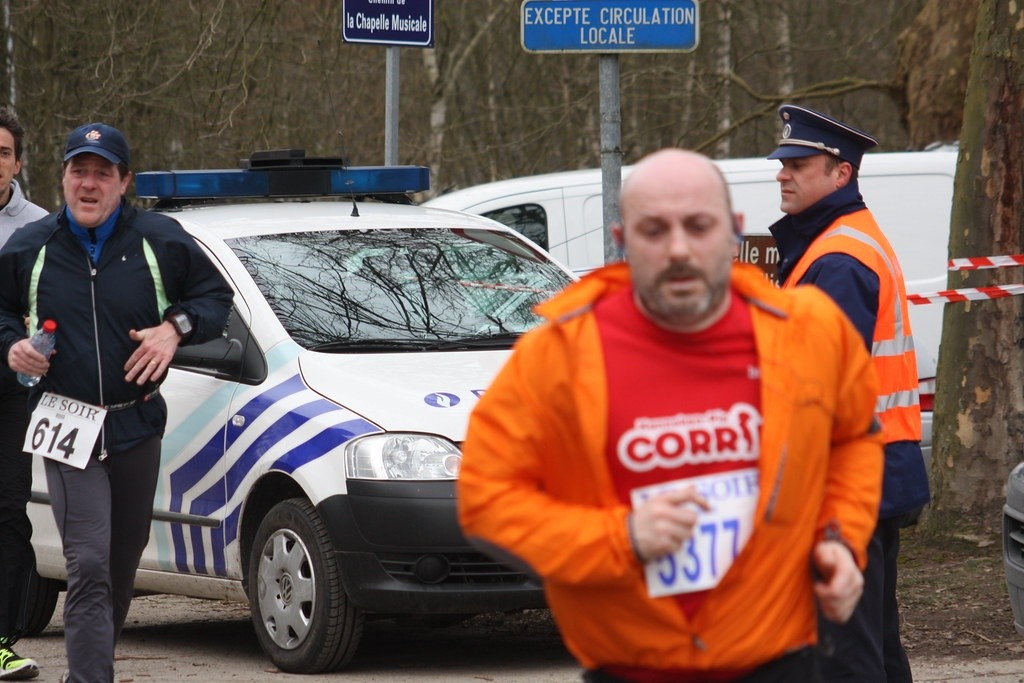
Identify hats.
[766,104,879,169]
[63,122,130,166]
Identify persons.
[454,149,885,683]
[1,122,235,683]
[1,110,52,680]
[767,96,935,683]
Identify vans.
[25,148,580,674]
[415,145,960,466]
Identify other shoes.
[61,668,70,683]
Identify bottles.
[16,320,57,387]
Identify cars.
[1001,460,1023,638]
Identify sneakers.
[0,630,39,679]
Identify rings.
[151,360,158,367]
